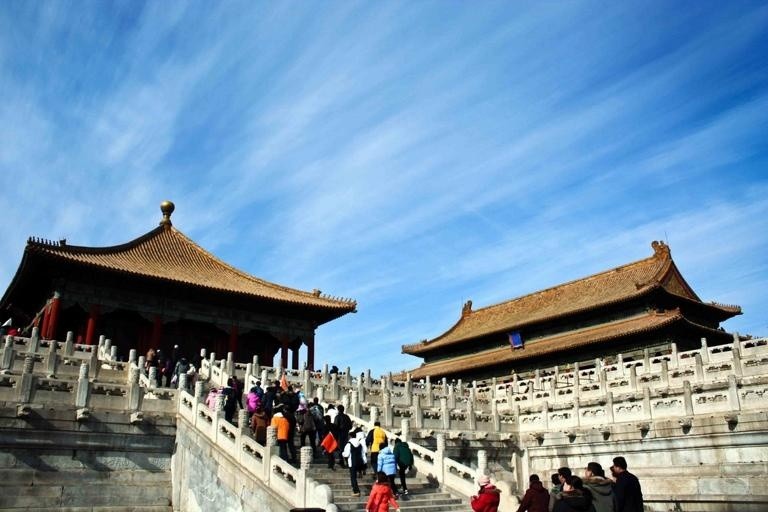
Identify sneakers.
[396,484,408,498]
[352,493,360,497]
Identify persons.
[470,455,642,512]
[0,322,22,337]
[145,344,416,512]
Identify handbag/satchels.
[369,502,378,512]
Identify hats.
[478,477,490,486]
